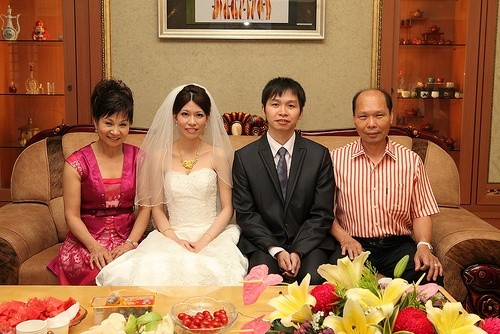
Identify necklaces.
[176,137,201,175]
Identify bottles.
[25,64,39,94]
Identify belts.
[354,236,408,246]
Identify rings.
[434,264,438,267]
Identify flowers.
[231,249,500,334]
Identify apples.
[124,311,163,334]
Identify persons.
[232,78,338,286]
[94,83,250,298]
[331,88,445,288]
[44,77,150,286]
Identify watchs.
[126,239,138,248]
[417,241,433,254]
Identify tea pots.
[0,4,21,40]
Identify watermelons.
[0,297,81,334]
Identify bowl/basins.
[17,118,40,148]
[90,293,156,327]
[170,295,238,334]
[69,305,88,328]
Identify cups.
[46,81,55,95]
[15,317,70,334]
[9,81,18,94]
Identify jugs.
[428,24,440,33]
[410,8,424,18]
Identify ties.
[276,147,287,203]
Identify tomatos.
[177,309,228,334]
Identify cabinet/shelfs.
[396,41,464,99]
[0,39,66,149]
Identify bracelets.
[203,232,213,240]
[162,228,174,235]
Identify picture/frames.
[157,0,324,41]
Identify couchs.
[0,113,500,321]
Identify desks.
[0,284,319,334]
